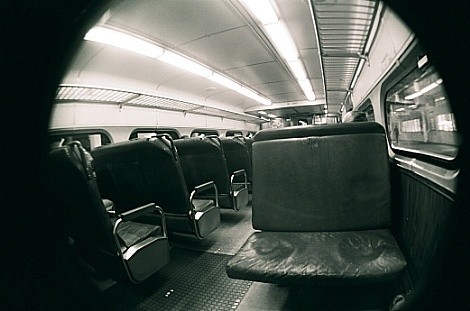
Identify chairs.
[47,119,408,281]
